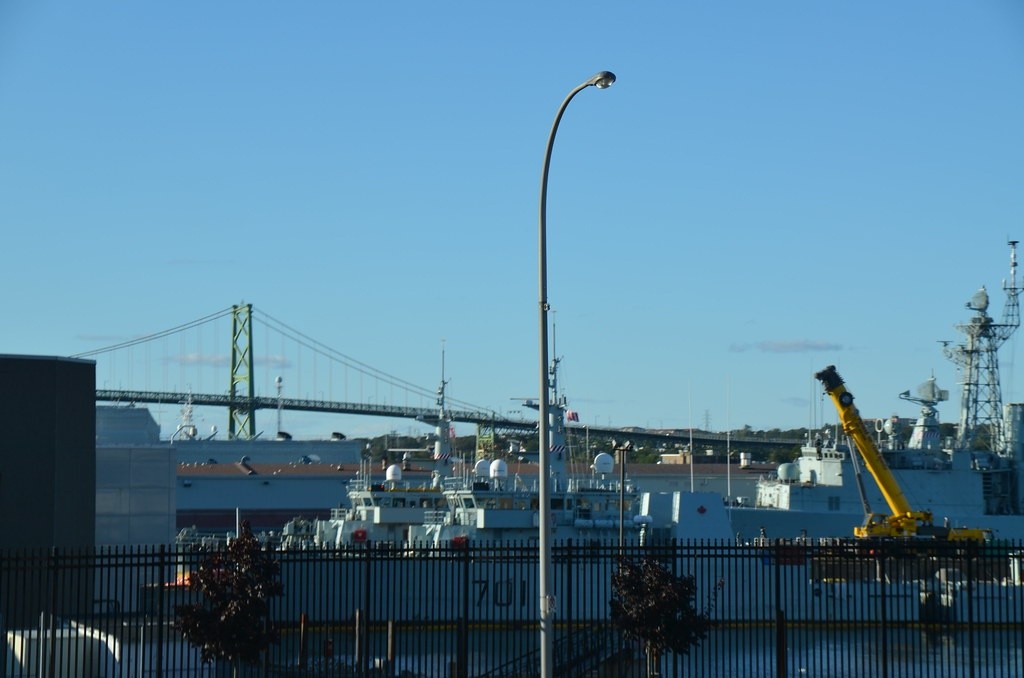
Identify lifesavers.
[354,530,366,541]
[501,498,512,509]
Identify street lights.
[538,67,619,678]
[610,438,634,572]
[273,376,281,440]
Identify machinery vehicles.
[810,365,995,558]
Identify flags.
[567,412,580,422]
